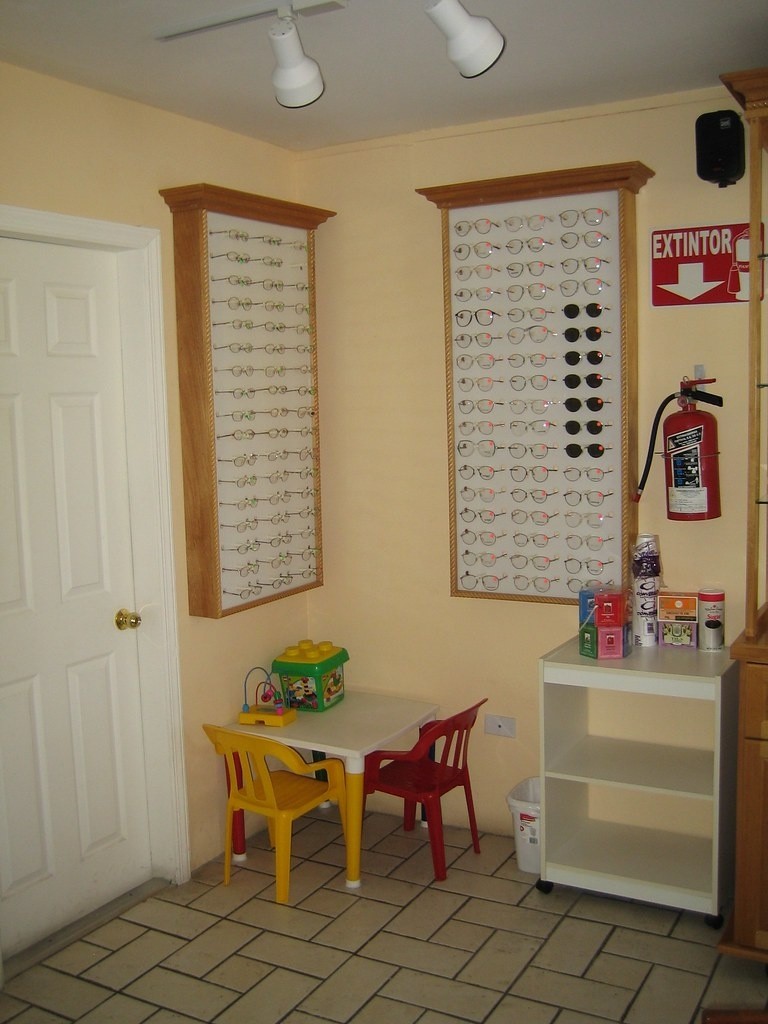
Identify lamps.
[423,0,506,80]
[267,19,325,109]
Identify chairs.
[202,723,347,904]
[361,697,488,882]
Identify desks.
[220,689,439,889]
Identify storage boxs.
[656,592,699,623]
[271,644,350,713]
[658,621,698,650]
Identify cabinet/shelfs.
[537,634,740,933]
[718,67,768,976]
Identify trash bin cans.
[505,775,541,874]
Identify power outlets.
[484,713,517,739]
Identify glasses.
[210,228,324,601]
[453,207,614,591]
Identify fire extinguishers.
[634,375,724,522]
